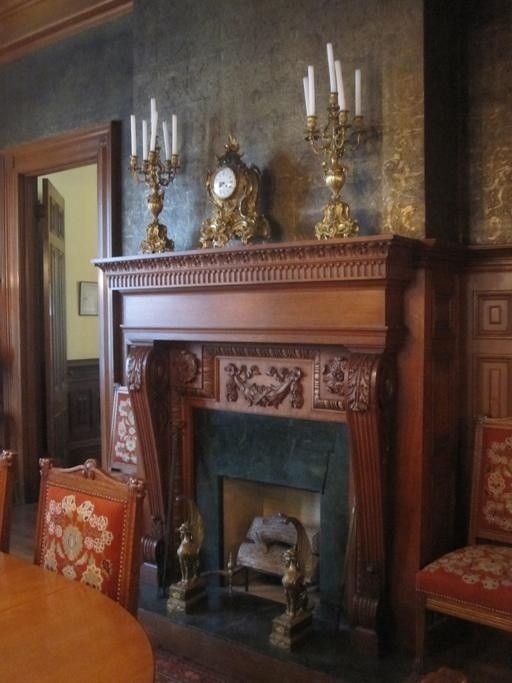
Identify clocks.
[193,127,276,251]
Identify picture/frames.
[78,279,100,317]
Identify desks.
[2,552,158,681]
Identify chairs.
[413,409,511,680]
[0,436,22,558]
[30,455,146,622]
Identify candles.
[125,90,184,163]
[293,41,371,119]
